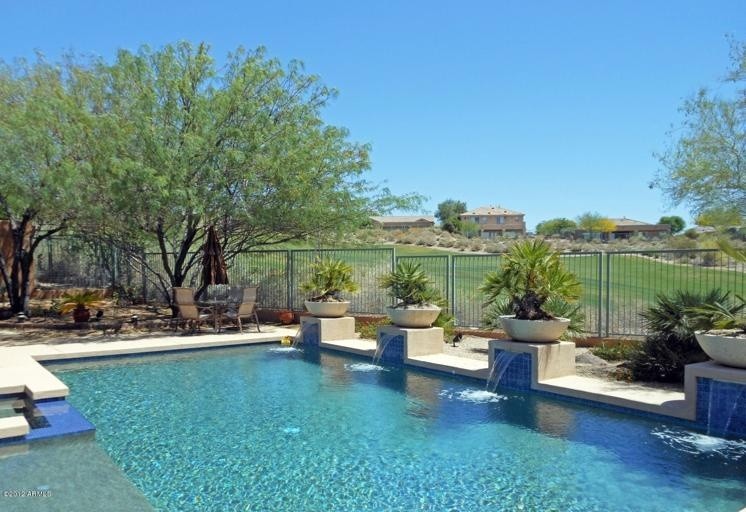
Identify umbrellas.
[200,226,229,331]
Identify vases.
[279,309,296,325]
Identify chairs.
[169,283,262,335]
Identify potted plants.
[679,291,746,368]
[57,289,101,324]
[300,236,583,340]
